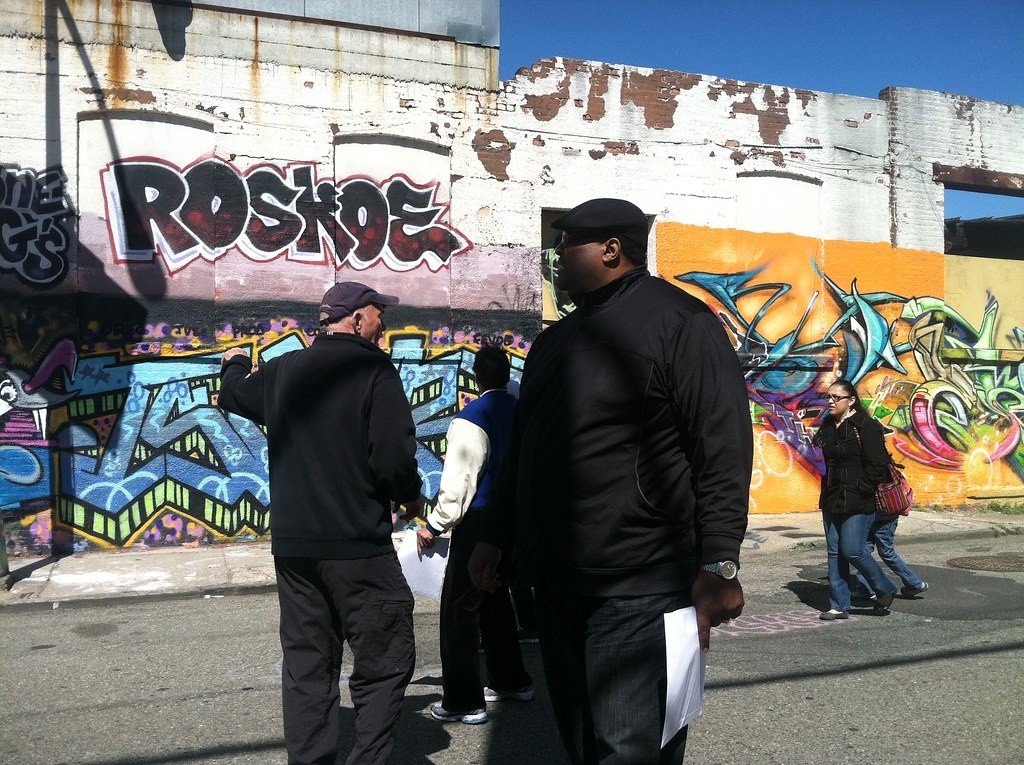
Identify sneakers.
[431,703,488,724]
[483,683,534,702]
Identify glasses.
[824,394,851,402]
[560,235,625,248]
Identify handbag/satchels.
[875,481,909,516]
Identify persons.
[217,282,424,765]
[417,348,540,726]
[850,456,929,601]
[469,199,752,765]
[818,379,897,621]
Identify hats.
[551,198,648,251]
[318,281,400,326]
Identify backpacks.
[889,465,913,517]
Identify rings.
[722,619,730,623]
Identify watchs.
[702,560,738,580]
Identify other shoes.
[819,611,848,620]
[901,581,928,596]
[517,628,539,643]
[478,635,485,654]
[872,593,895,616]
[851,591,876,600]
